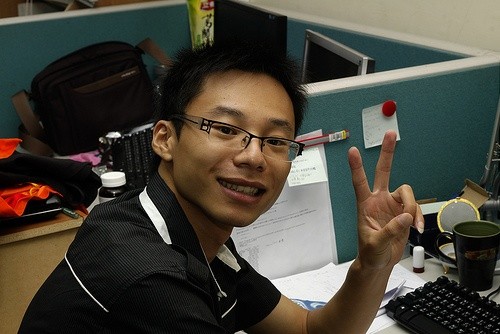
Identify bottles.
[99,172,128,204]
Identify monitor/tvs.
[299,28,375,85]
[214,0,288,54]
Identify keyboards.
[384,275,500,334]
[111,125,160,195]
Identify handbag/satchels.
[31,41,157,157]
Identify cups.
[434,220,500,292]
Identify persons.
[16,37,425,333]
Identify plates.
[438,242,456,268]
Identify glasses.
[166,108,305,162]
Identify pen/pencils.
[60,204,80,219]
